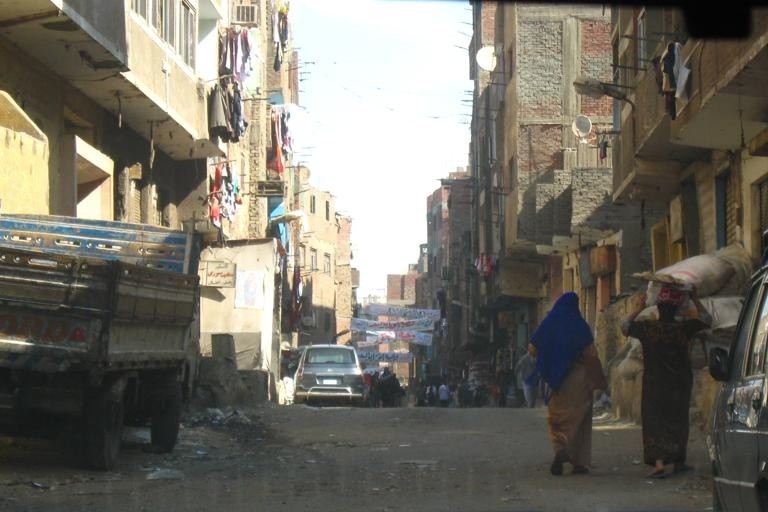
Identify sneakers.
[551,448,563,475]
[676,463,694,473]
[574,466,593,474]
[646,467,667,479]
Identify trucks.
[0,207,203,470]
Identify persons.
[527,292,615,477]
[622,282,714,477]
[279,337,551,411]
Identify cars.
[702,263,768,512]
[292,342,369,407]
